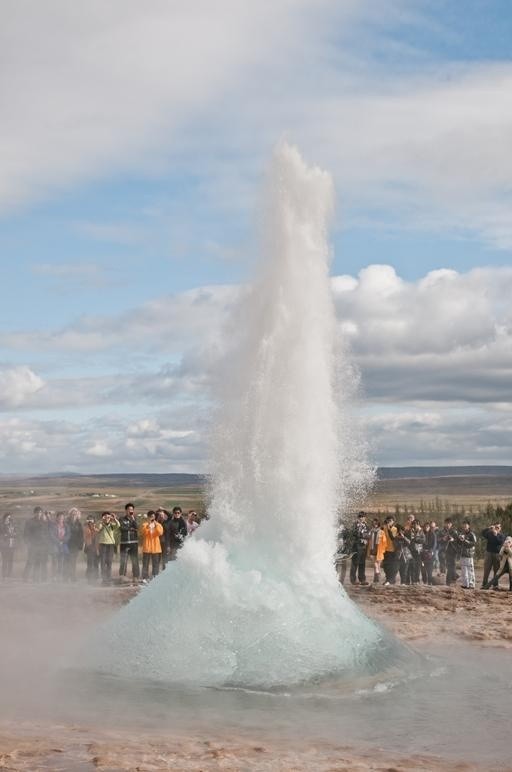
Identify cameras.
[151,519,154,521]
[489,526,494,529]
[192,513,196,515]
[107,515,111,518]
[458,535,466,538]
[87,520,92,523]
[384,520,387,523]
[129,512,133,514]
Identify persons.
[332,511,512,592]
[0,504,209,586]
